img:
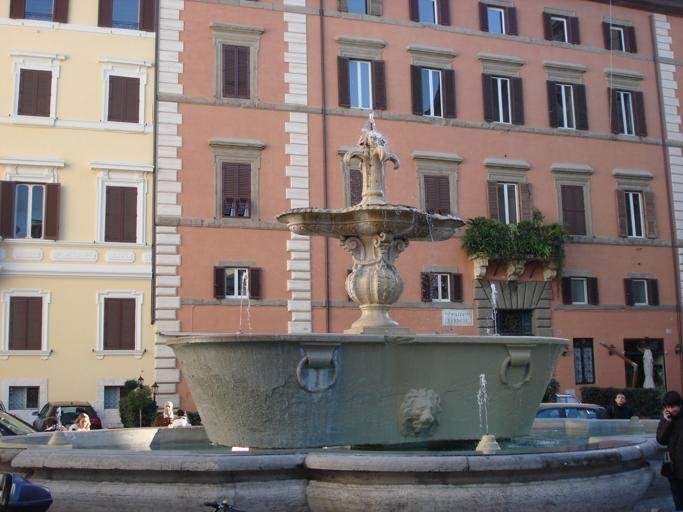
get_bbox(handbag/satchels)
[660,461,675,478]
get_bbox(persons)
[69,412,91,431]
[605,393,633,419]
[657,391,683,512]
[167,410,192,427]
[153,401,178,426]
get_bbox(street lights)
[151,380,158,404]
[137,374,146,427]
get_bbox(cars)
[554,393,581,402]
[534,402,608,419]
[31,401,100,430]
